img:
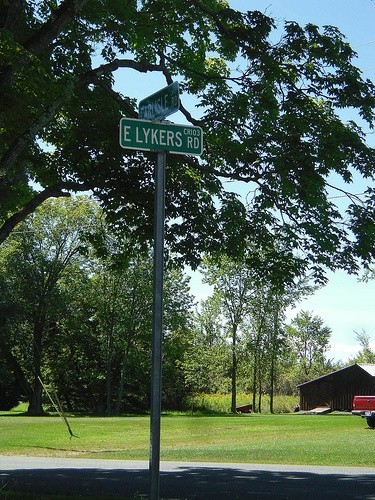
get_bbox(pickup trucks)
[352,394,375,427]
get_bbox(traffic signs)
[120,117,203,156]
[139,81,180,122]
